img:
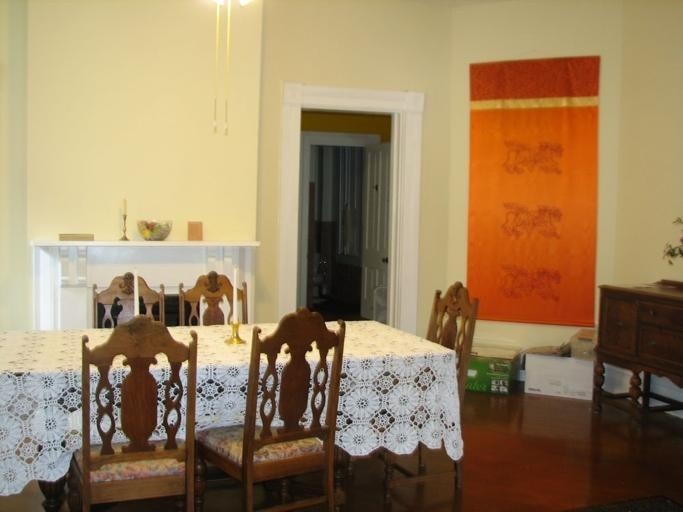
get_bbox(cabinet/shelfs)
[31,238,262,331]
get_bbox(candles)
[121,198,127,216]
[231,267,239,324]
[131,269,140,317]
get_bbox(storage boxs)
[568,326,597,361]
[466,345,520,397]
[523,345,594,400]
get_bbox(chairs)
[69,311,198,511]
[346,277,482,505]
[90,271,165,330]
[176,271,249,328]
[195,306,350,509]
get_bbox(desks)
[0,316,466,511]
[590,274,682,447]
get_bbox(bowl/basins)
[137,221,173,241]
